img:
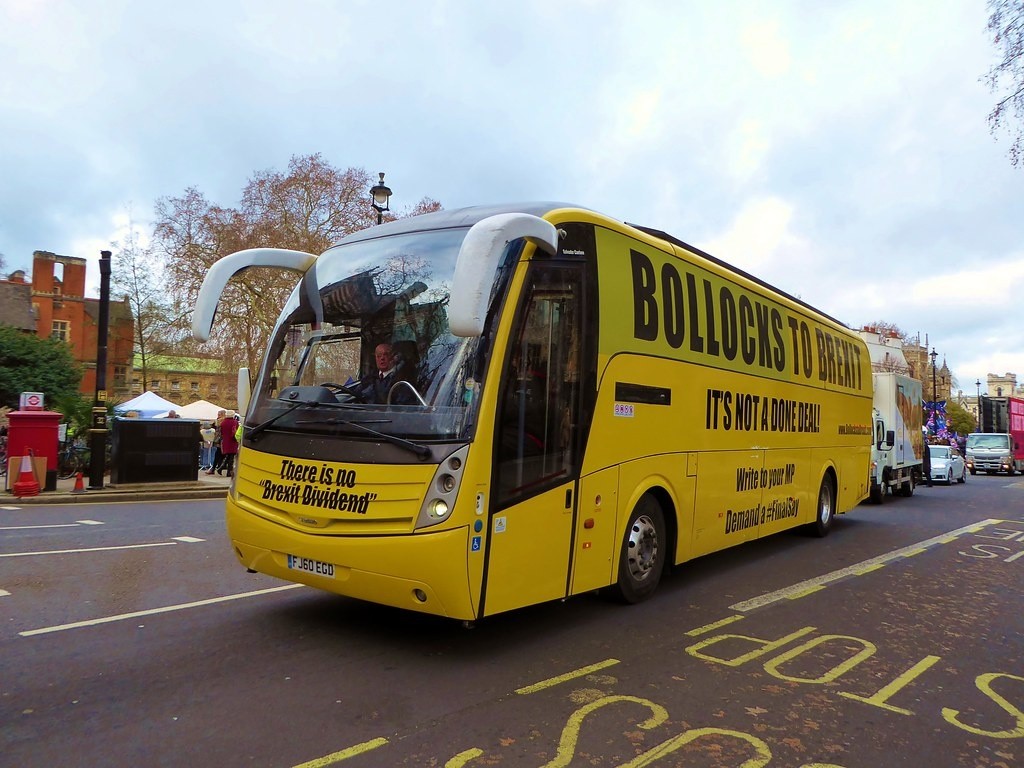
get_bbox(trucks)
[871,370,923,503]
[965,395,1024,477]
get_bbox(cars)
[918,444,967,485]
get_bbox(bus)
[187,198,877,629]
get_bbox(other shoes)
[228,473,233,477]
[923,484,933,488]
[205,467,215,474]
[217,469,223,475]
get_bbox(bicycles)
[56,441,90,480]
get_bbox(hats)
[225,410,235,417]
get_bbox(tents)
[106,391,181,423]
[152,399,240,420]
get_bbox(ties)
[380,372,384,381]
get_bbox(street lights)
[929,347,938,445]
[369,171,393,225]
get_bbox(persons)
[916,442,933,487]
[205,410,242,477]
[126,411,138,418]
[198,421,217,470]
[164,409,180,418]
[353,343,416,405]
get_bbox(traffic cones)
[12,445,39,496]
[72,473,87,492]
